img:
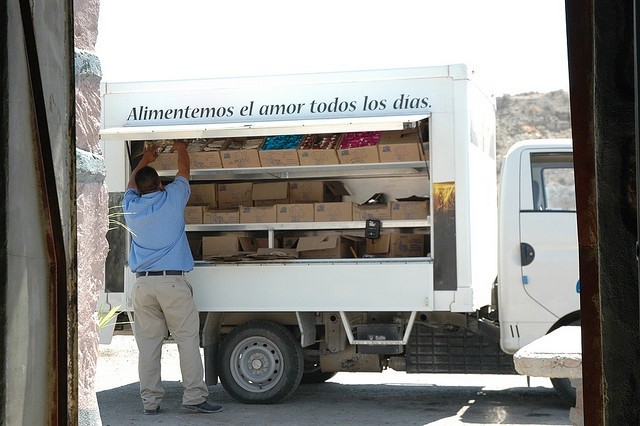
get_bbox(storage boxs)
[240,205,277,223]
[418,123,429,160]
[296,235,365,258]
[391,200,430,220]
[337,131,380,164]
[203,209,239,224]
[352,201,391,220]
[283,236,297,248]
[289,180,351,203]
[277,203,314,223]
[220,137,262,168]
[297,133,340,166]
[252,182,288,206]
[201,236,257,260]
[147,144,178,170]
[218,183,253,209]
[258,135,300,167]
[186,137,223,168]
[391,233,430,258]
[314,202,352,222]
[190,184,215,205]
[366,234,390,258]
[184,206,204,224]
[255,237,279,250]
[379,131,421,162]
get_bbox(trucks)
[100,64,580,407]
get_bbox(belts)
[137,270,183,276]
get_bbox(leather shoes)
[183,400,223,413]
[146,406,160,413]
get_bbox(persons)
[120,139,224,415]
